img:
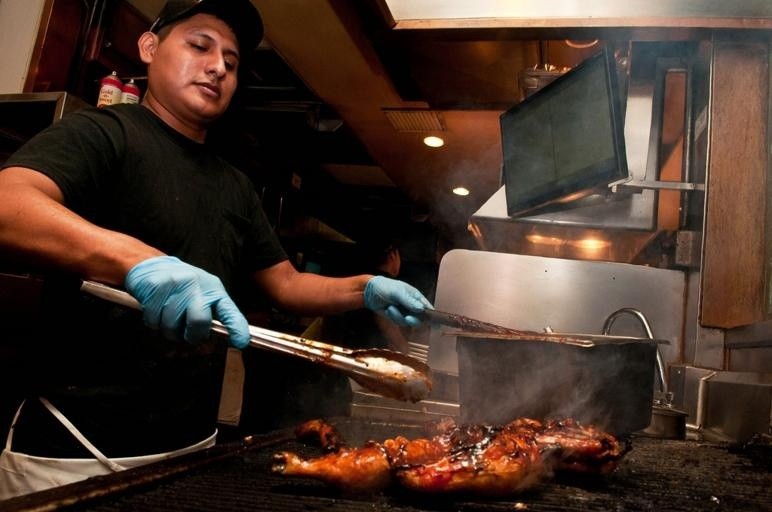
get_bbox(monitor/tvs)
[499,46,628,218]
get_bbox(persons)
[1,0,441,503]
[320,230,413,356]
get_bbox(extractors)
[467,41,657,265]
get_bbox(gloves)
[125,255,250,350]
[362,275,435,329]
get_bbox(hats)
[149,1,264,56]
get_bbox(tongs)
[76,273,435,405]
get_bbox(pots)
[442,324,670,444]
